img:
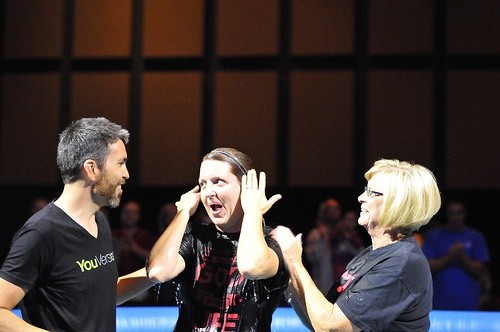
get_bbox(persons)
[268,156,441,332]
[0,117,158,332]
[145,147,291,332]
[26,198,492,313]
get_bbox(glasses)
[364,187,383,198]
